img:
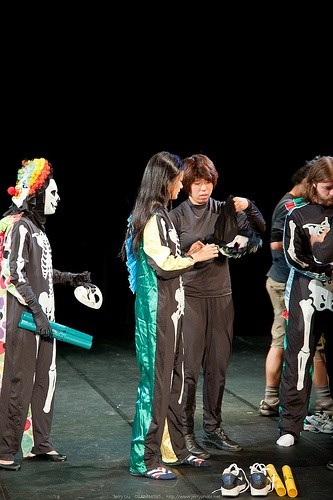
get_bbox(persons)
[276,157,333,447]
[257,156,333,419]
[167,152,269,465]
[120,150,212,482]
[0,153,95,472]
